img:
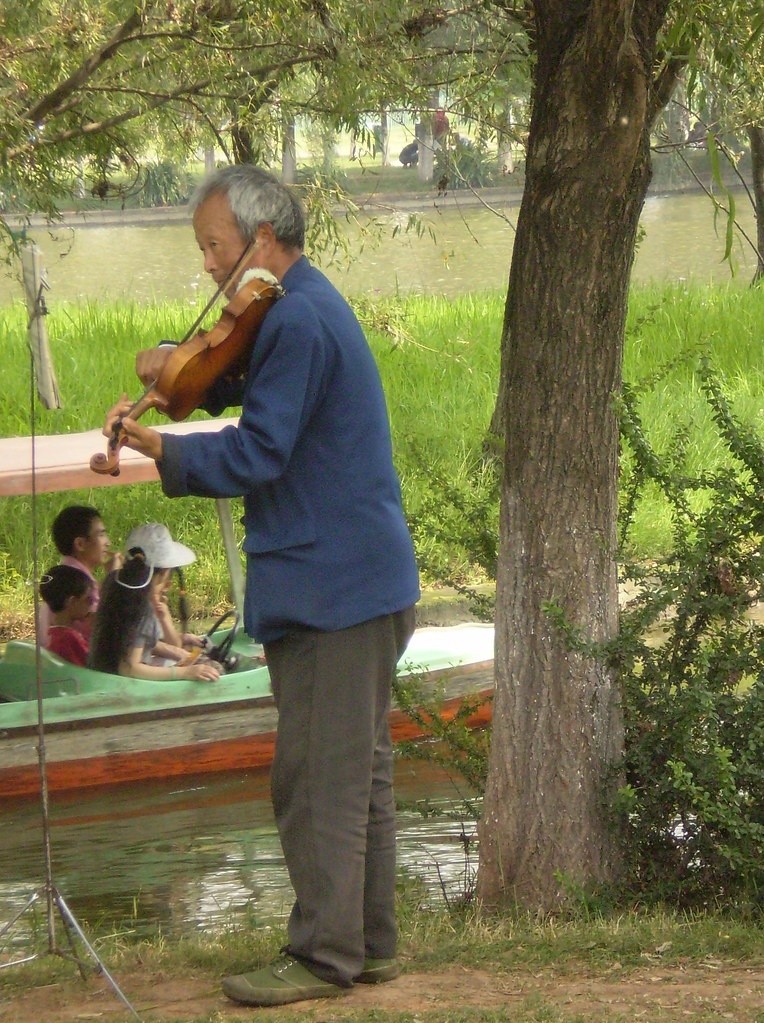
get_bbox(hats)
[125,523,195,569]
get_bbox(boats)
[0,417,495,796]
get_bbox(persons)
[398,139,419,169]
[39,506,224,684]
[104,164,421,1009]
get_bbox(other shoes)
[352,956,399,983]
[222,944,352,1004]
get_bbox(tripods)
[0,283,145,1023]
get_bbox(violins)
[88,277,281,479]
